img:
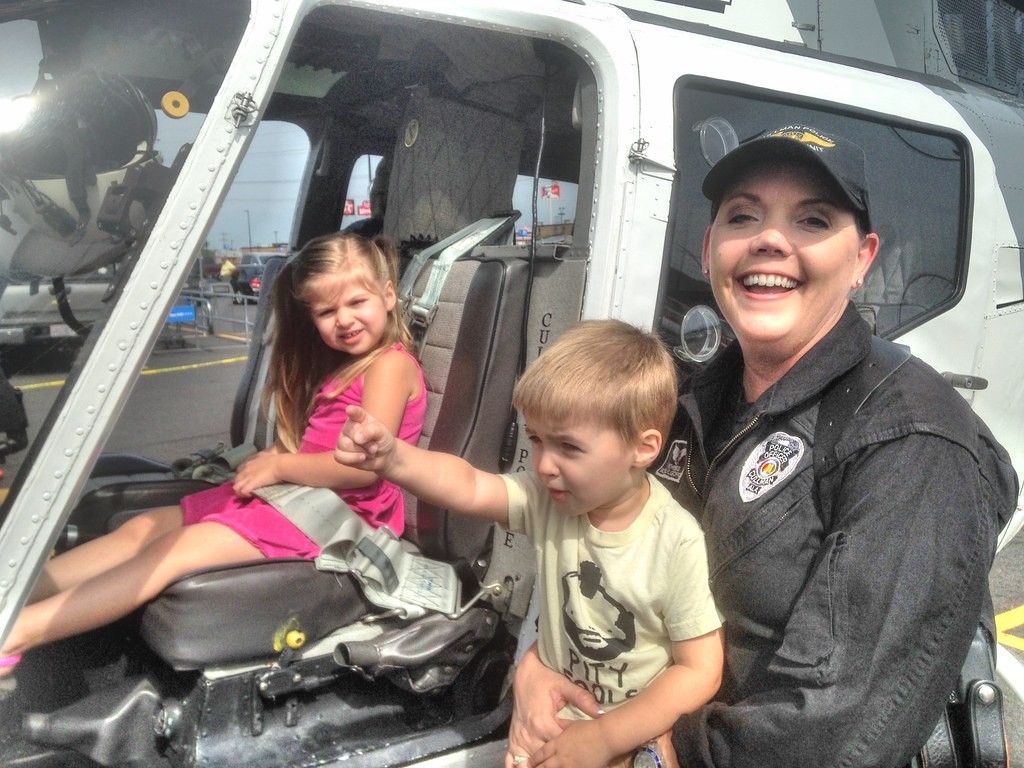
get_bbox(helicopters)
[0,0,1024,768]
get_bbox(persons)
[0,230,427,677]
[333,319,723,768]
[504,124,1020,768]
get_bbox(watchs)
[632,737,666,768]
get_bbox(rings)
[513,755,529,765]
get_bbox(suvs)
[231,253,282,304]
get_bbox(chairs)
[107,249,530,670]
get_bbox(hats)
[702,123,875,234]
